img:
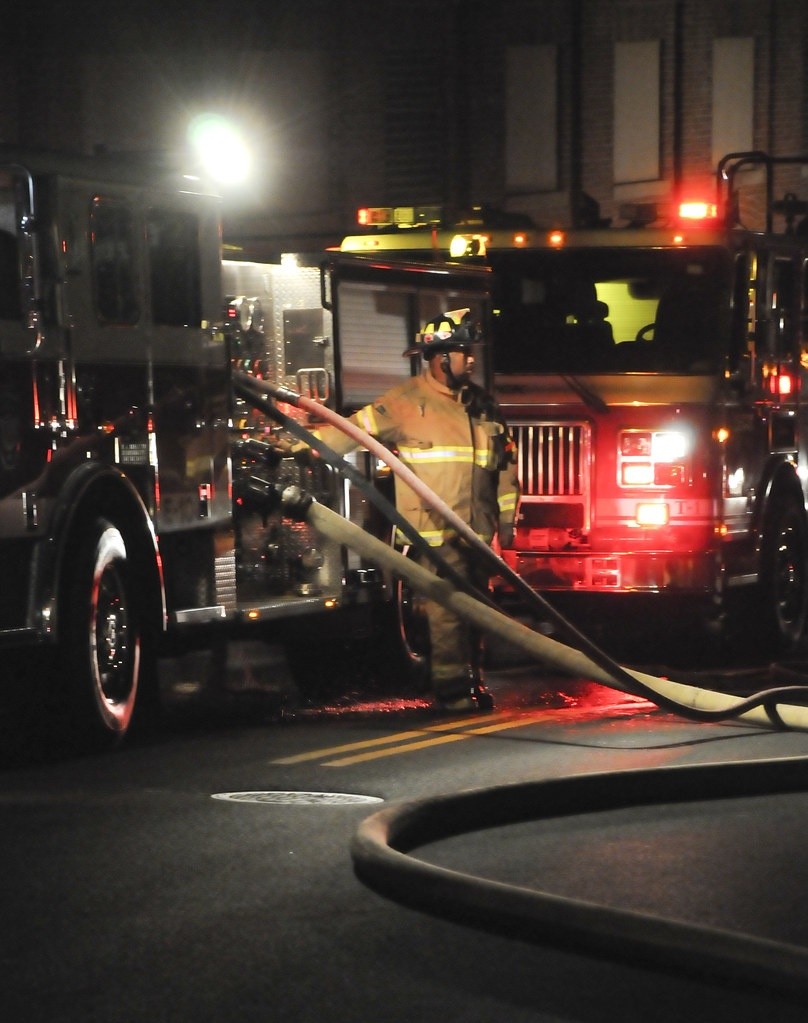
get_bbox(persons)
[276,308,521,714]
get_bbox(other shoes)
[473,676,496,711]
[432,688,474,714]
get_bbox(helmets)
[420,308,477,361]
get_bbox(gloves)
[498,522,513,550]
[267,438,293,458]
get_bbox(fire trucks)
[0,152,808,749]
